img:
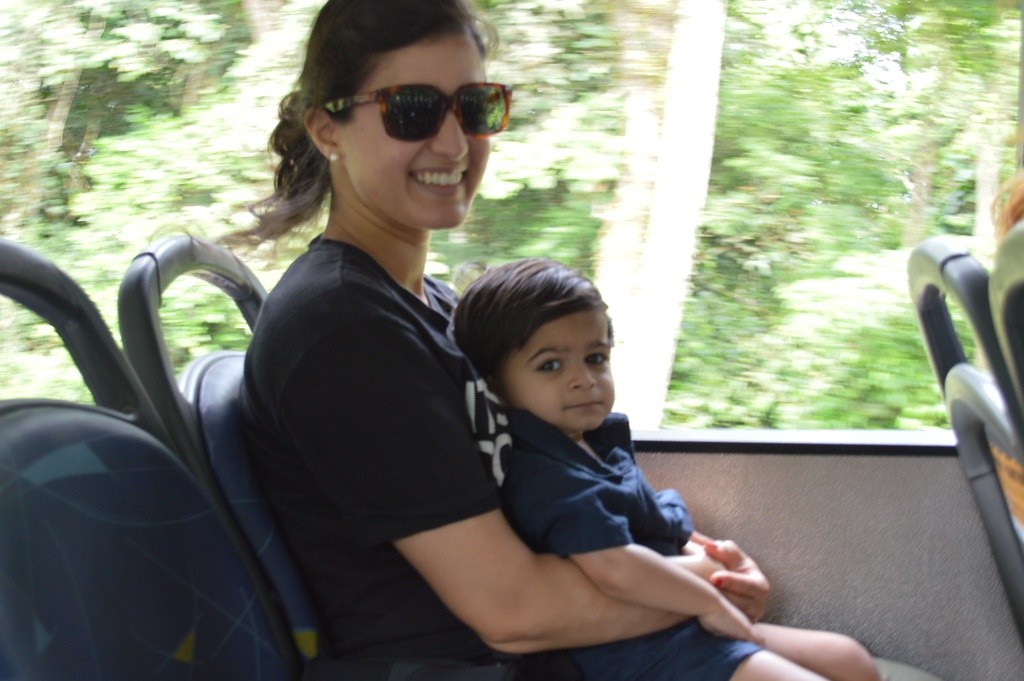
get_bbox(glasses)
[323,82,513,143]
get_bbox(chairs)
[117,235,334,681]
[905,232,1024,645]
[0,236,303,681]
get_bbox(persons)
[244,0,882,680]
